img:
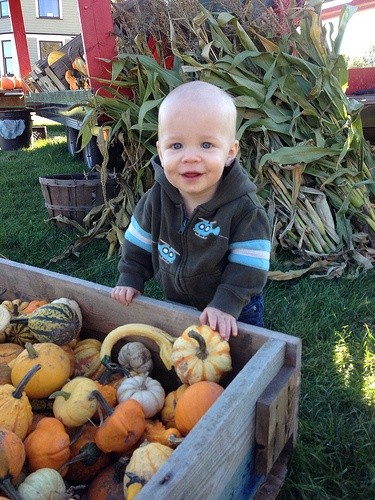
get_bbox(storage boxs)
[22,33,84,90]
[0,257,301,500]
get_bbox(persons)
[111,81,272,342]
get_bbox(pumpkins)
[0,298,232,500]
